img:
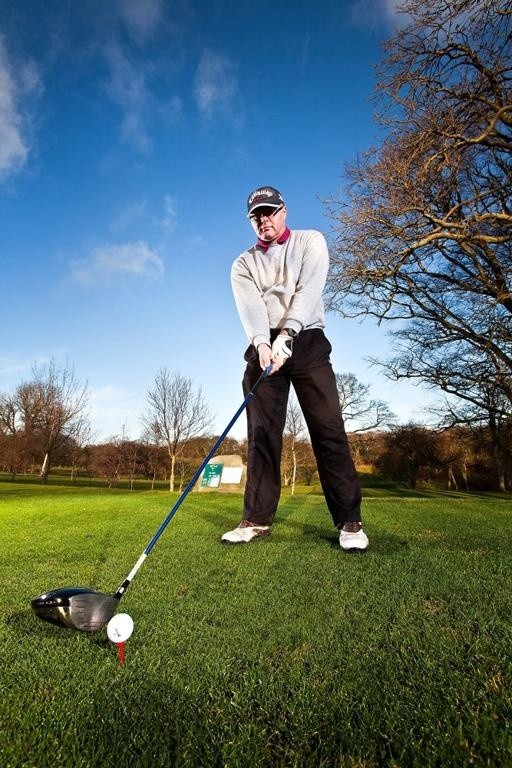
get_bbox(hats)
[247,187,283,218]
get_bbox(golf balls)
[106,613,134,643]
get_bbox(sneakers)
[221,520,271,545]
[338,521,368,551]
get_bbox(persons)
[220,185,371,551]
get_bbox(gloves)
[257,335,293,374]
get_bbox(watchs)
[284,328,297,339]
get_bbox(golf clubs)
[33,359,273,633]
